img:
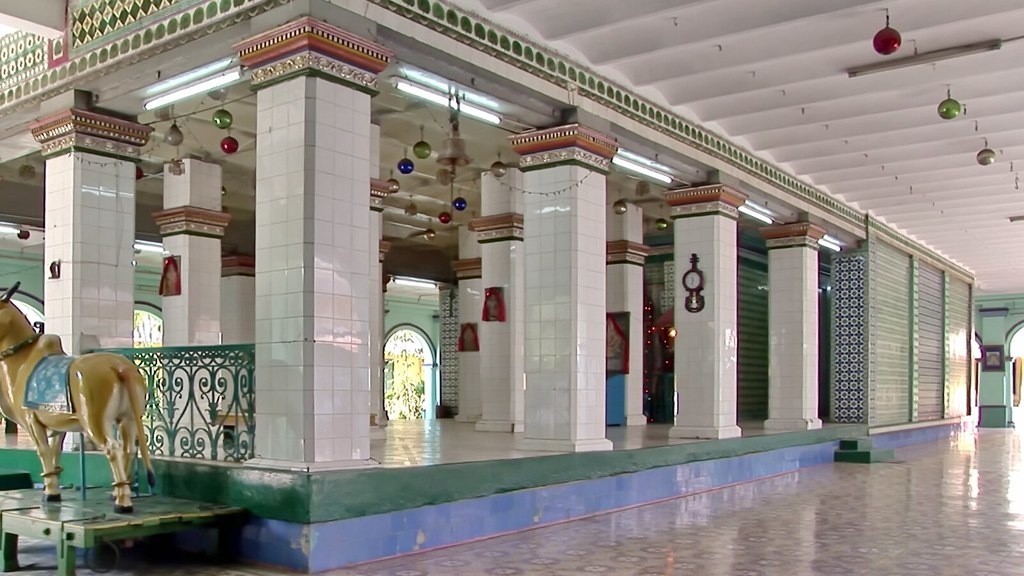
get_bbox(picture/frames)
[981,345,1006,372]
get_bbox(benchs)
[217,413,256,449]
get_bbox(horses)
[0,279,158,516]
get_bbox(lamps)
[142,65,242,111]
[612,189,668,230]
[937,87,961,119]
[738,205,775,224]
[847,38,1002,77]
[389,76,503,125]
[976,140,995,166]
[166,101,239,154]
[385,125,507,240]
[612,154,675,183]
[872,8,901,56]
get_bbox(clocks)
[682,253,705,313]
